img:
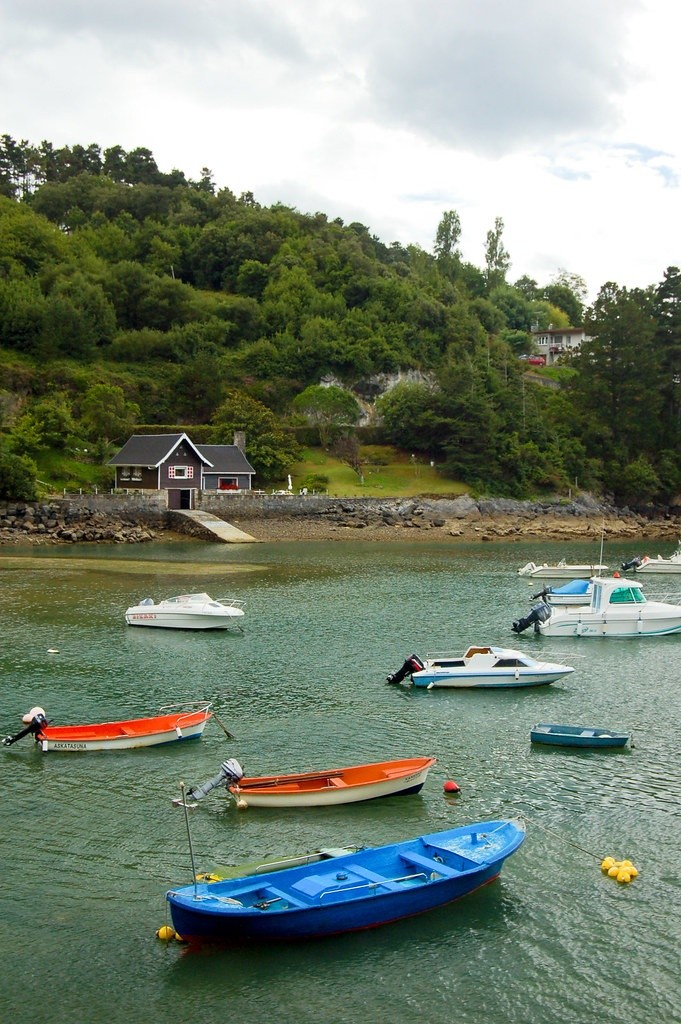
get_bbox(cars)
[519,354,546,366]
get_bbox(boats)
[1,699,215,753]
[530,722,629,748]
[512,576,681,638]
[528,517,641,605]
[387,646,578,688]
[125,593,246,632]
[186,756,438,810]
[620,540,681,573]
[517,557,608,578]
[165,817,526,949]
